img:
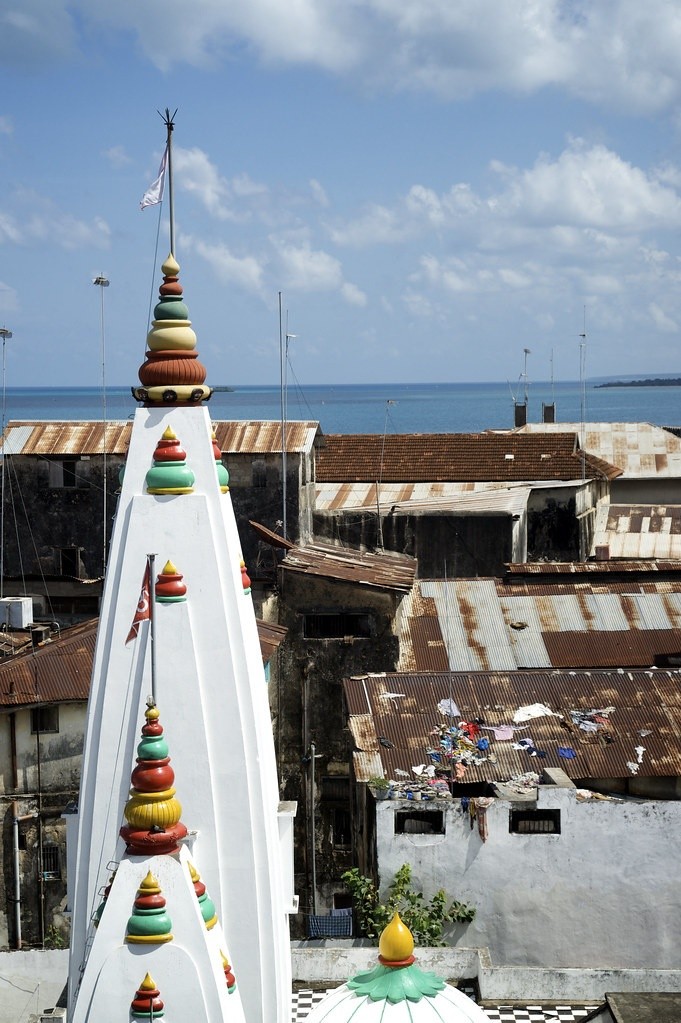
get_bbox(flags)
[126,559,152,643]
[140,149,167,209]
